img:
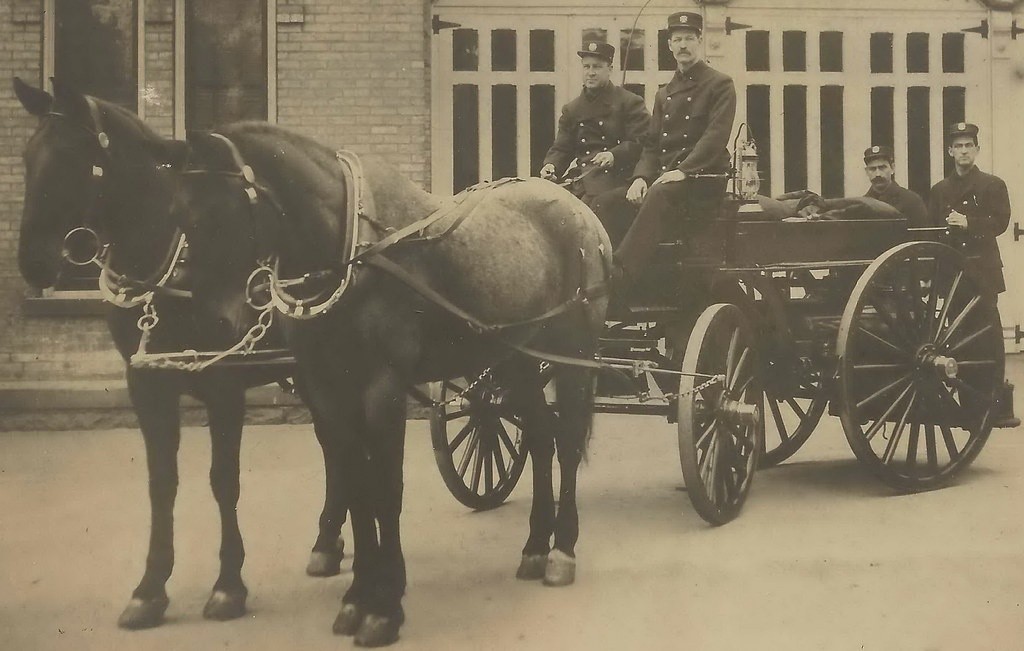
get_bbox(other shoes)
[612,261,638,298]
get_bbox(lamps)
[733,123,761,201]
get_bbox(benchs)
[632,194,742,266]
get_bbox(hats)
[864,146,893,165]
[577,40,615,64]
[667,12,703,35]
[949,122,979,137]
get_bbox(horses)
[143,120,615,648]
[8,72,345,631]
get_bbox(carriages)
[10,73,1024,646]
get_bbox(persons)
[539,41,653,251]
[590,12,737,287]
[862,123,1011,407]
